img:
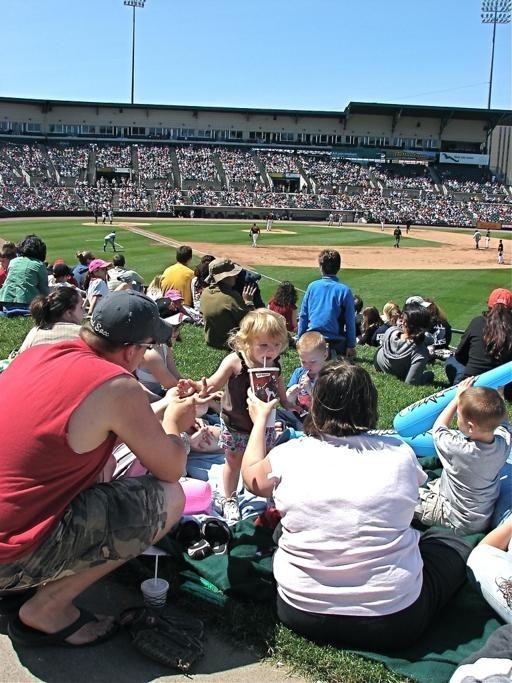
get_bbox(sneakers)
[219,497,242,520]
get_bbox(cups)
[139,578,169,611]
[248,367,279,428]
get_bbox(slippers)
[3,602,118,646]
[176,515,234,557]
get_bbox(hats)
[90,290,186,344]
[202,256,246,283]
[487,285,512,308]
[52,264,72,277]
[405,293,434,308]
[87,260,114,272]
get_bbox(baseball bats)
[114,242,124,249]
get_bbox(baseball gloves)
[120,604,205,673]
